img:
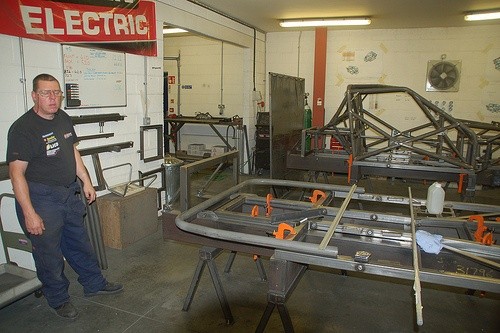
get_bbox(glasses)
[36,90,63,97]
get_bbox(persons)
[7,74,124,320]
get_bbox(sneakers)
[84,282,124,296]
[48,301,79,320]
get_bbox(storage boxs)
[211,146,228,157]
[187,143,204,155]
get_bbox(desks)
[164,116,243,170]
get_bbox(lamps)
[280,17,372,27]
[464,10,500,21]
[163,26,189,34]
